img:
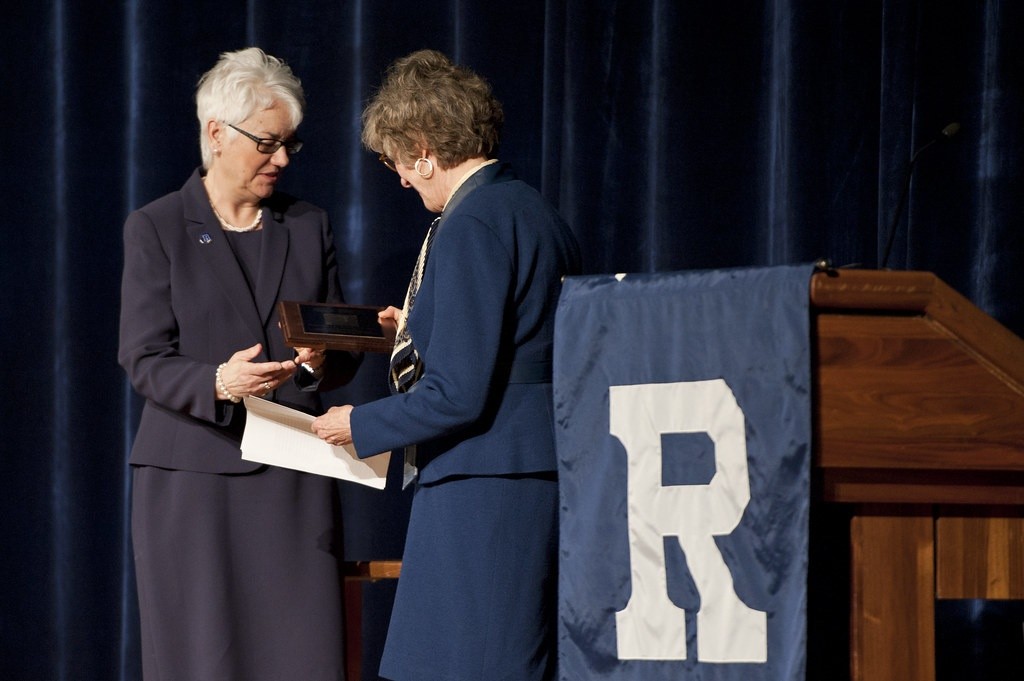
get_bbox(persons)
[112,46,367,681]
[309,51,572,681]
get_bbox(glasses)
[378,152,399,172]
[227,124,303,154]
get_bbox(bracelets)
[215,362,245,403]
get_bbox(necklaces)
[200,177,264,232]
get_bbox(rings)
[263,382,274,391]
